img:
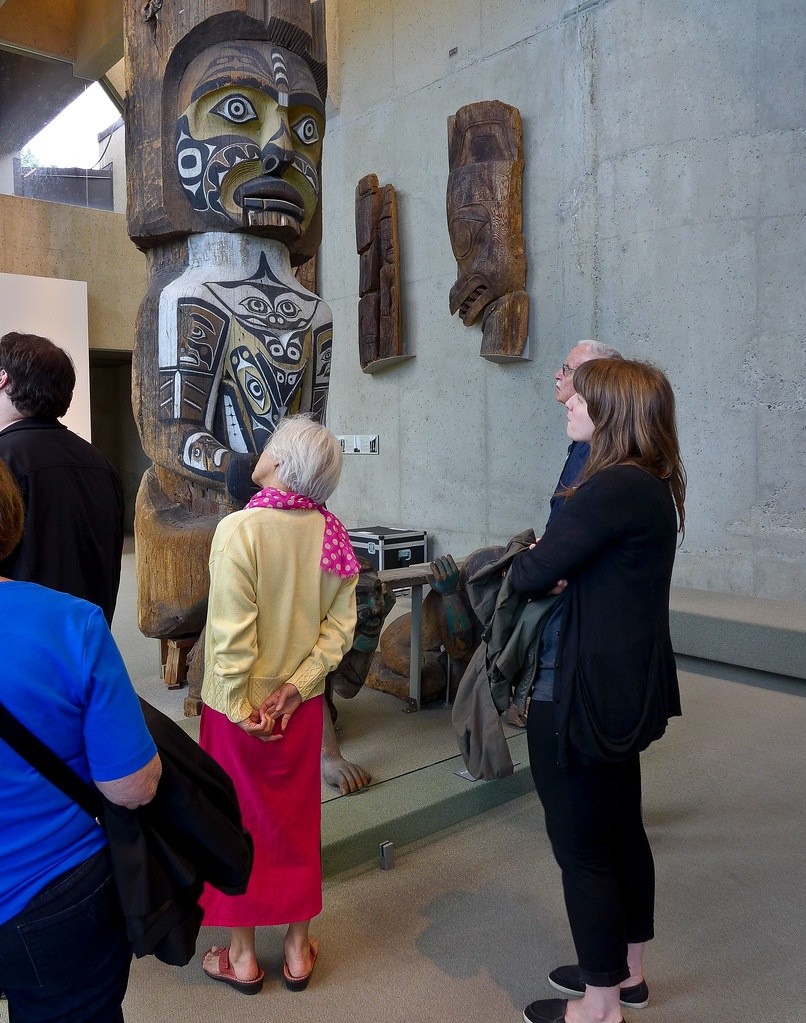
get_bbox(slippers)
[201,945,265,995]
[283,934,319,992]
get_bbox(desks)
[380,568,464,710]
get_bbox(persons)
[198,420,359,996]
[125,0,373,795]
[545,339,623,504]
[0,331,126,628]
[0,462,163,1023]
[511,359,684,1022]
[364,545,506,704]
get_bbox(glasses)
[562,364,576,376]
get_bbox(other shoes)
[548,965,650,1008]
[523,998,626,1023]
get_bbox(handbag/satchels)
[108,810,205,969]
